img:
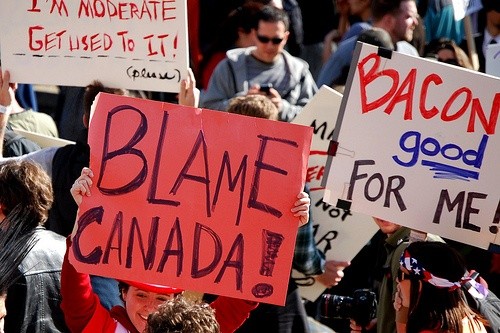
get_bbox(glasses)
[256,32,285,46]
[394,14,419,21]
[436,56,456,65]
[396,268,410,282]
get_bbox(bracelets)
[0,105,12,114]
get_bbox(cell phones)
[260,84,272,97]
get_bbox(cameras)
[317,289,377,328]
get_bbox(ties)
[489,38,498,44]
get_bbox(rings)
[76,180,80,184]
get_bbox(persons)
[0,68,200,235]
[6,83,59,138]
[61,167,311,333]
[227,96,351,333]
[202,7,318,123]
[394,242,488,333]
[142,294,220,333]
[0,160,71,333]
[223,0,500,89]
